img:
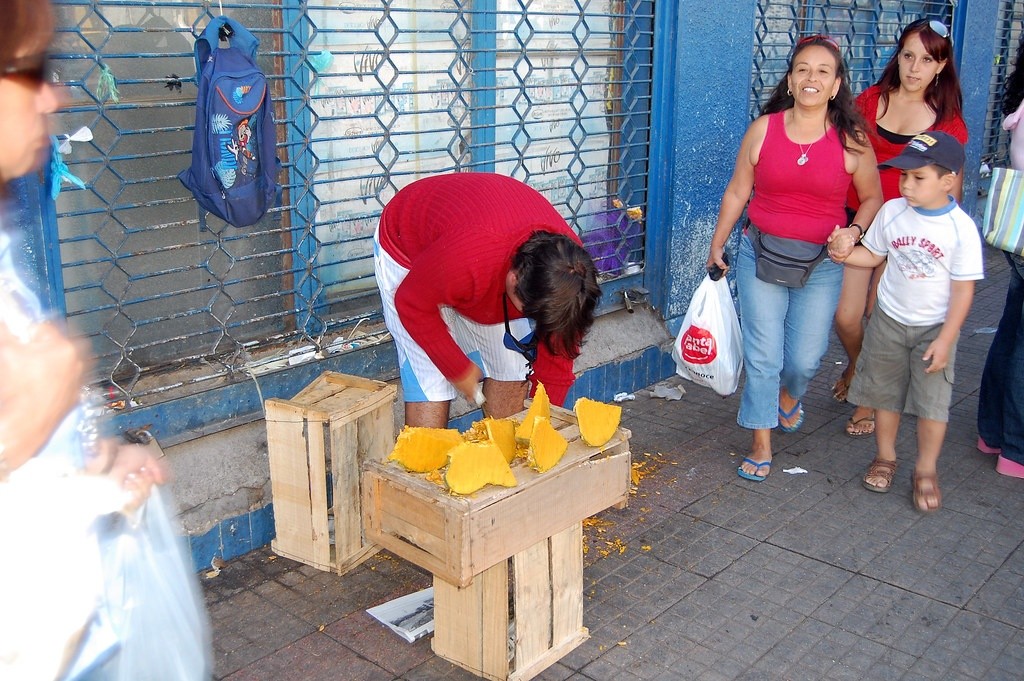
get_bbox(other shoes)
[977,436,1001,454]
[995,454,1024,478]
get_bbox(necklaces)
[794,127,821,165]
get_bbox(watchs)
[848,224,864,243]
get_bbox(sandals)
[911,466,942,512]
[862,459,897,493]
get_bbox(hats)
[877,130,965,174]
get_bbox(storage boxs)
[431,522,590,681]
[265,370,397,576]
[361,397,632,589]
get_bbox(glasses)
[0,50,48,90]
[904,19,950,38]
[799,36,839,53]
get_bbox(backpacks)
[176,15,282,228]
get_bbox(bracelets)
[98,440,118,475]
[0,444,12,484]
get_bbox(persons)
[706,33,884,482]
[372,173,602,431]
[830,18,966,436]
[0,0,165,681]
[825,130,983,508]
[976,44,1024,479]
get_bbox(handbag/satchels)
[746,223,830,288]
[982,167,1024,257]
[120,468,209,681]
[671,273,743,396]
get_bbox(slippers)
[738,458,771,481]
[778,401,805,433]
[846,417,875,439]
[835,371,849,402]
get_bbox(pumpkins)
[528,416,569,472]
[514,380,550,446]
[483,417,516,464]
[388,424,464,472]
[444,442,517,494]
[574,396,622,445]
